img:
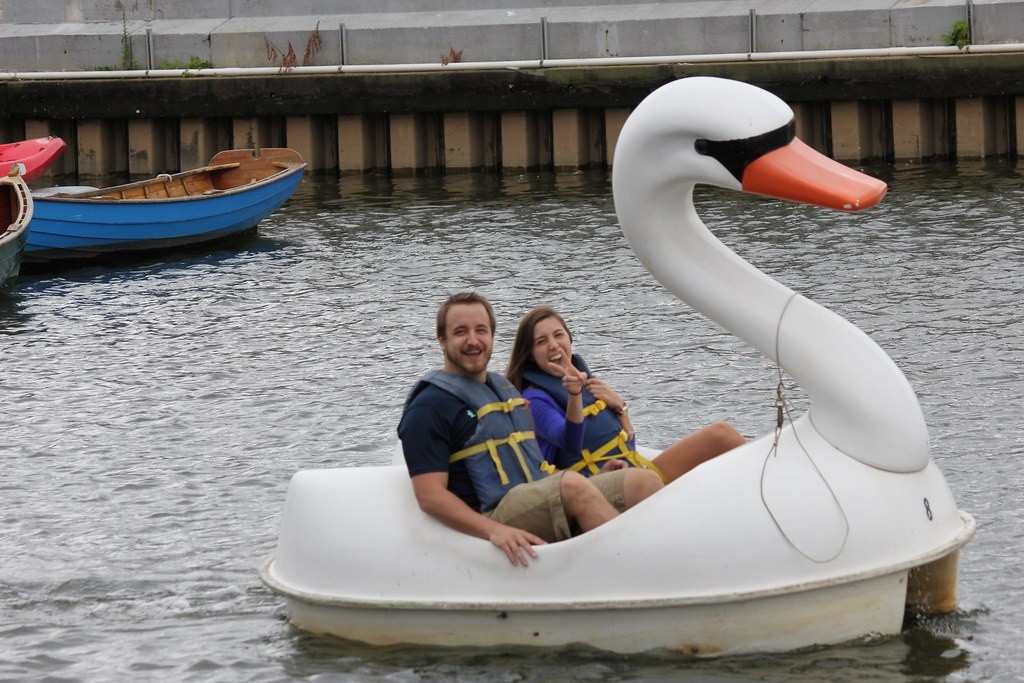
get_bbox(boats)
[0,163,34,287]
[21,146,308,253]
[258,75,978,655]
[0,135,66,185]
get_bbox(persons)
[506,306,748,486]
[396,292,665,567]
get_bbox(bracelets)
[568,388,582,395]
[618,402,629,416]
